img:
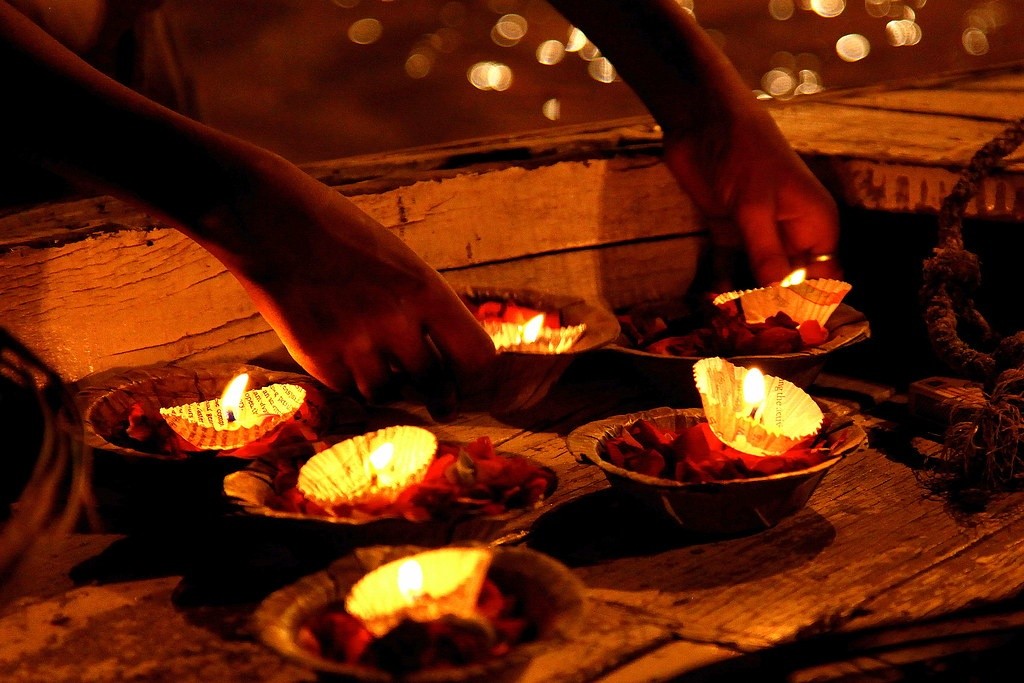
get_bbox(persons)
[0,2,845,404]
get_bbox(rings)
[807,253,839,263]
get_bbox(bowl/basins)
[597,299,871,393]
[399,286,620,414]
[57,360,298,465]
[220,435,560,548]
[564,407,867,528]
[250,544,588,683]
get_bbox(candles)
[738,408,762,424]
[364,472,392,491]
[407,591,433,608]
[221,408,239,431]
